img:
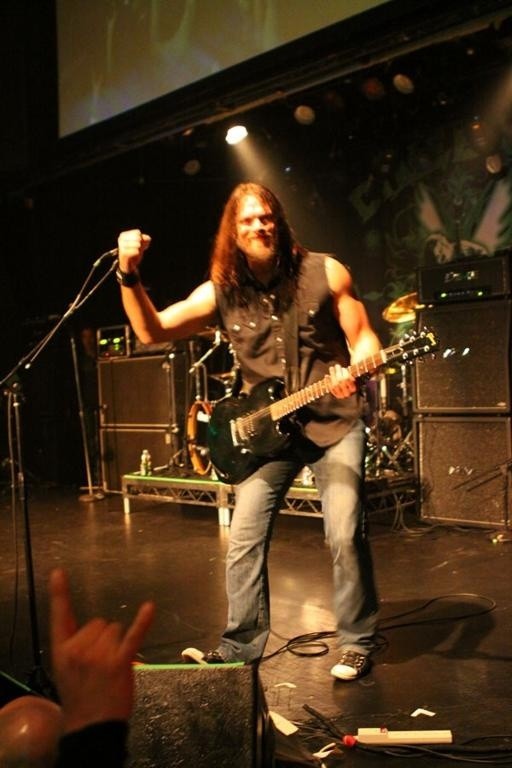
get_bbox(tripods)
[363,365,415,476]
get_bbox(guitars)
[207,329,440,485]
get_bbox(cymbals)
[382,292,419,323]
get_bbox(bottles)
[139,449,152,477]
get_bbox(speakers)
[99,427,186,494]
[96,353,187,428]
[128,661,277,768]
[411,416,512,532]
[410,299,511,413]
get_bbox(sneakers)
[330,650,374,681]
[180,645,226,665]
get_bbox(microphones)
[91,404,108,410]
[215,324,221,345]
[196,447,210,457]
[101,242,150,257]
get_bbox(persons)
[48,567,157,768]
[0,695,64,768]
[110,179,386,682]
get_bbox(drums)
[377,366,410,421]
[185,400,226,476]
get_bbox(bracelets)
[114,268,141,287]
